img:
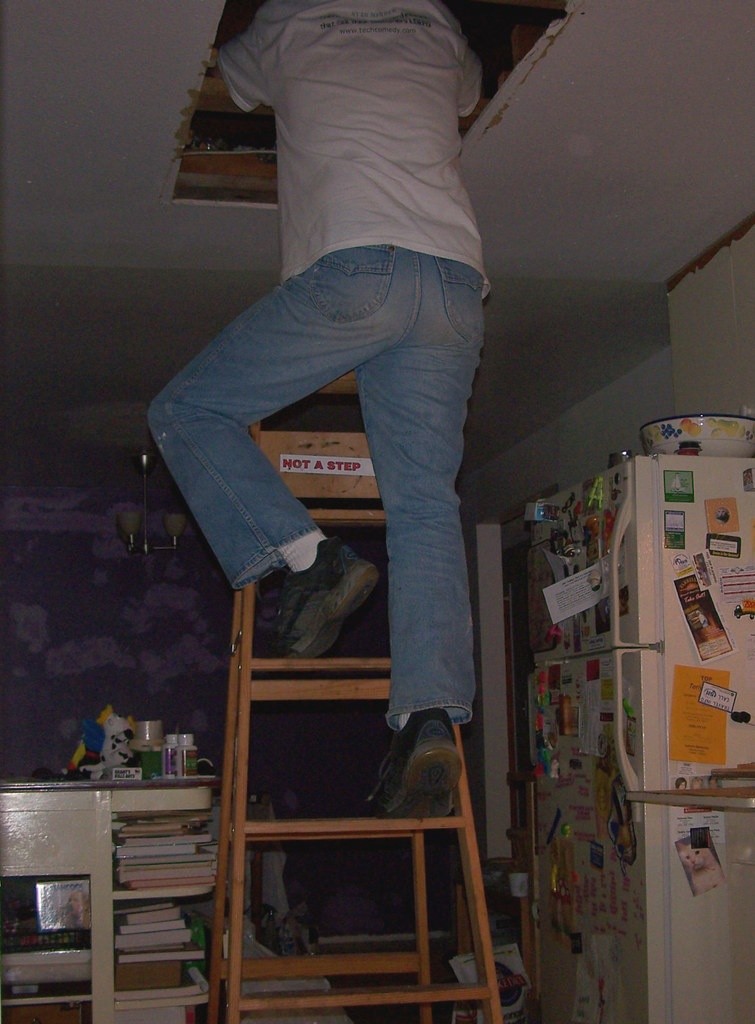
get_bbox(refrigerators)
[525,452,755,1023]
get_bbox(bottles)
[161,733,199,780]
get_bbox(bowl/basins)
[639,414,755,456]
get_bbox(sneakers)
[364,708,461,848]
[262,537,379,660]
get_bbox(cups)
[608,449,632,468]
[509,871,529,899]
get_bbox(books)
[112,817,218,1024]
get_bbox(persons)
[148,0,494,821]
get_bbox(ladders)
[207,366,510,1024]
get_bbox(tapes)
[133,720,164,740]
